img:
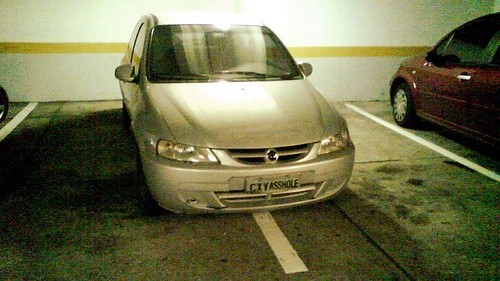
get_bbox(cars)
[114,12,354,214]
[390,12,500,163]
